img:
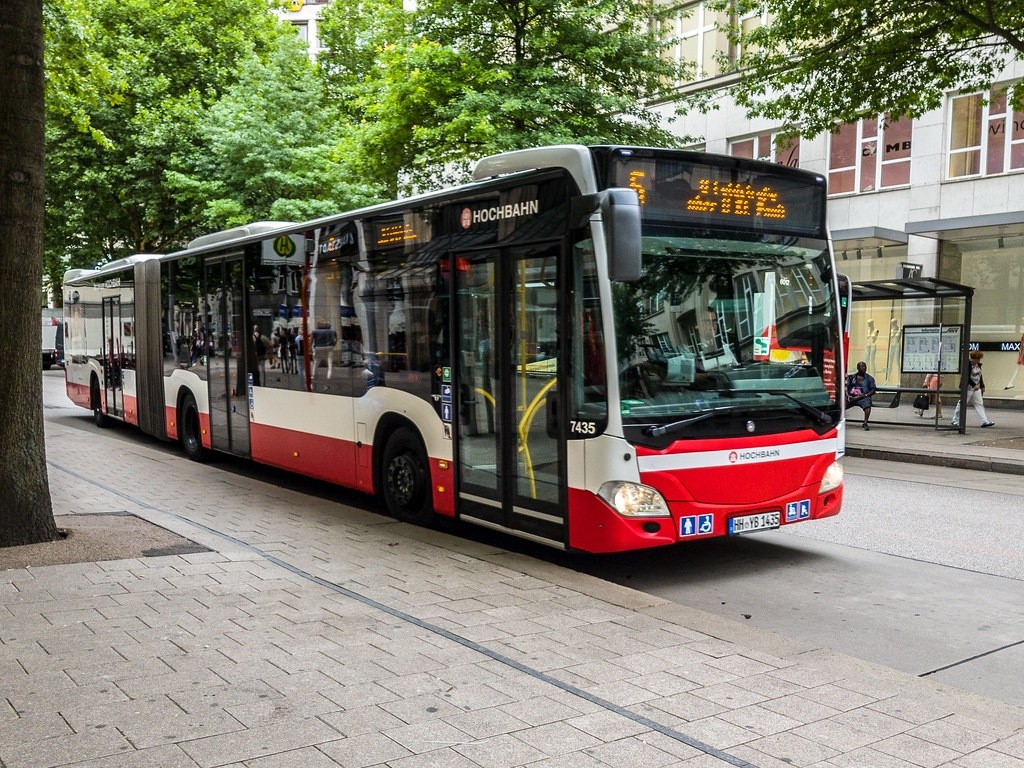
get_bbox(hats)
[254,325,260,332]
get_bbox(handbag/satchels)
[849,387,863,397]
[914,393,929,410]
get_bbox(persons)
[695,307,743,370]
[863,318,901,386]
[181,317,338,385]
[844,361,877,431]
[951,350,995,430]
[1003,332,1024,389]
[582,314,603,386]
[912,372,943,421]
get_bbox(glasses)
[703,319,718,326]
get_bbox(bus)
[63,144,852,552]
[753,264,852,405]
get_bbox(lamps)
[856,249,861,260]
[998,237,1005,249]
[877,247,882,258]
[841,251,848,261]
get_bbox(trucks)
[41,309,64,370]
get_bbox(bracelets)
[865,394,868,397]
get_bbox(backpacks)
[254,334,266,356]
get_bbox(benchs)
[870,389,901,408]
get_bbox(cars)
[54,322,64,366]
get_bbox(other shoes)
[291,370,298,375]
[271,366,275,369]
[282,368,285,374]
[981,422,994,427]
[862,423,869,430]
[286,367,289,373]
[278,363,280,369]
[931,415,942,420]
[914,409,923,416]
[951,422,960,427]
